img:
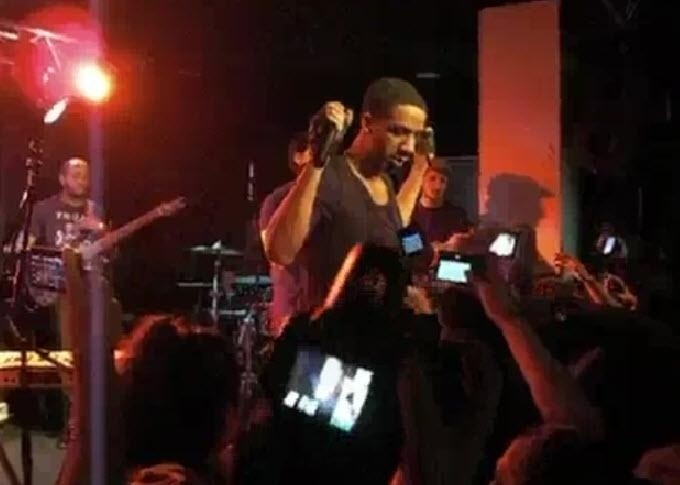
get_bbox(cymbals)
[177,282,222,288]
[182,246,244,256]
[226,275,271,285]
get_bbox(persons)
[8,154,123,449]
[57,78,680,485]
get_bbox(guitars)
[16,196,187,313]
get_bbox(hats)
[431,159,450,174]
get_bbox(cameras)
[437,250,476,287]
[597,227,619,259]
[397,224,424,255]
[487,227,519,261]
[286,338,376,438]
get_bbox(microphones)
[246,156,256,201]
